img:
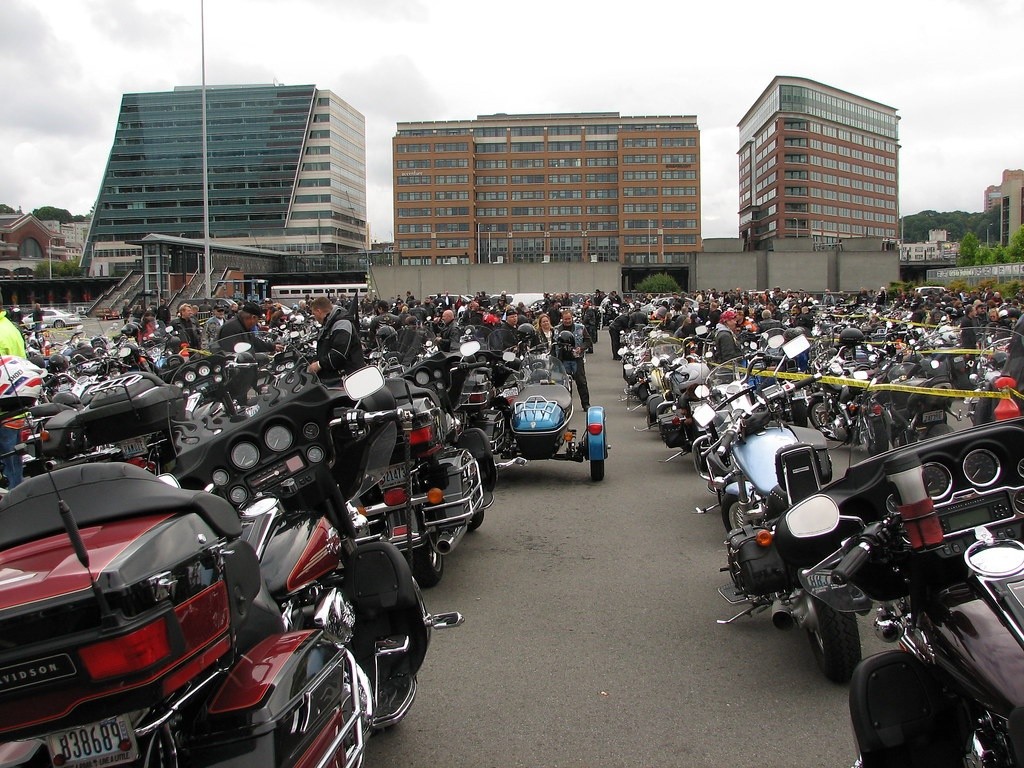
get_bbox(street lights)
[48,237,56,279]
[793,218,798,238]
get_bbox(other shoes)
[586,350,593,353]
[584,405,591,411]
[613,356,622,360]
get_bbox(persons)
[0,281,1024,491]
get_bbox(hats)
[214,305,224,310]
[243,302,261,317]
[506,309,518,320]
[719,311,737,324]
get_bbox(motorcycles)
[623,294,1024,767]
[1,313,613,768]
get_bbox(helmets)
[498,295,507,301]
[46,354,69,373]
[783,326,805,344]
[120,323,139,337]
[839,328,865,345]
[376,326,398,347]
[0,355,48,413]
[518,323,536,339]
[557,329,576,352]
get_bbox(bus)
[271,284,367,310]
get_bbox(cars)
[915,287,945,302]
[19,310,82,330]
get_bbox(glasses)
[981,308,986,309]
[217,310,224,312]
